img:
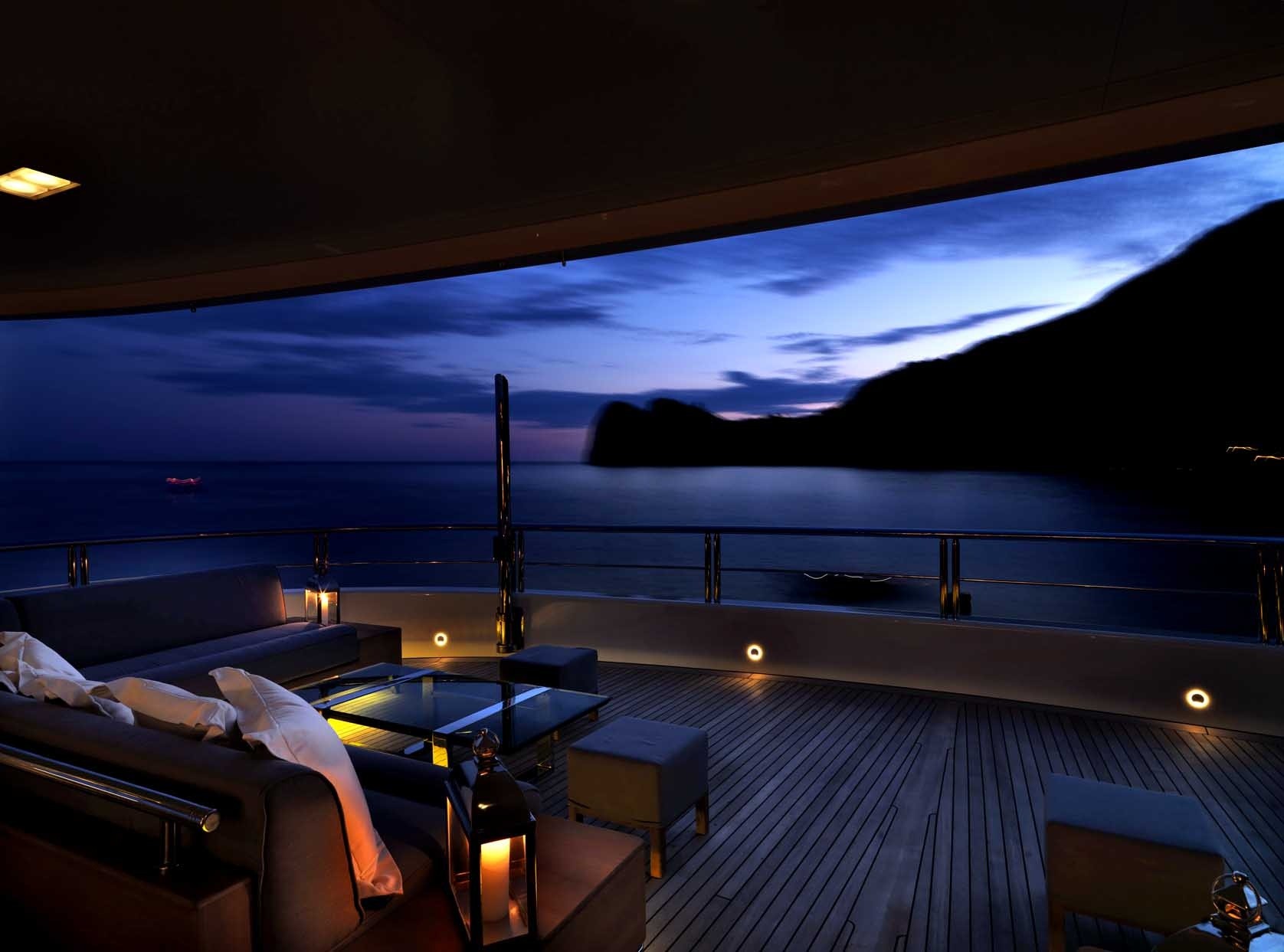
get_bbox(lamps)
[443,730,538,951]
[306,566,342,628]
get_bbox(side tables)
[290,617,402,669]
[344,813,645,951]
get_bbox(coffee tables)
[297,664,610,775]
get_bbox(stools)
[500,646,600,741]
[1043,771,1227,952]
[565,715,709,879]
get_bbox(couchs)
[0,565,356,709]
[0,685,540,952]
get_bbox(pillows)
[90,674,235,742]
[1,630,94,707]
[205,668,403,900]
